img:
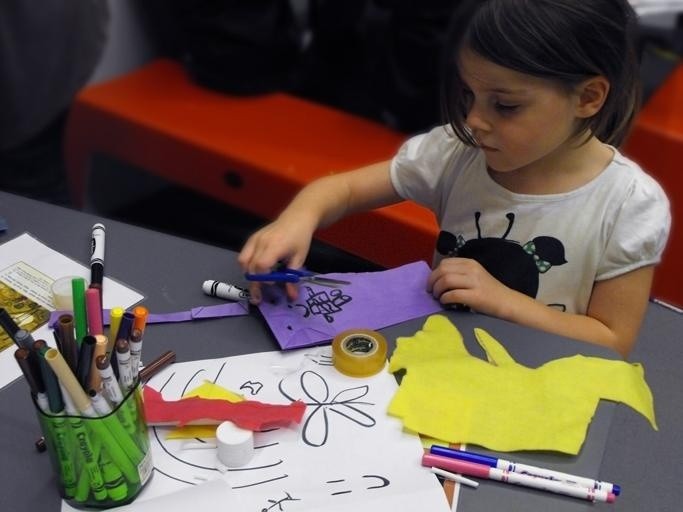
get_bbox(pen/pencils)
[2,219,149,498]
[420,442,622,506]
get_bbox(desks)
[610,64,682,309]
[1,186,683,512]
[63,53,446,274]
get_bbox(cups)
[29,375,154,509]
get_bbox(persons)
[238,0,672,362]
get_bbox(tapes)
[332,325,389,377]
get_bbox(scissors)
[241,263,352,299]
